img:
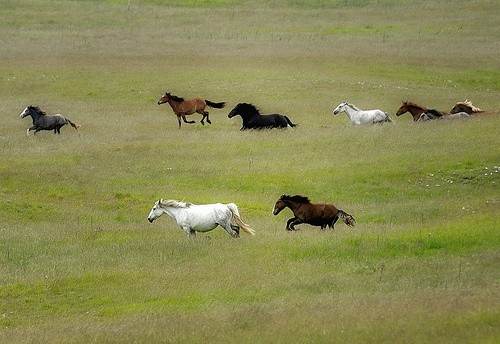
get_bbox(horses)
[148,199,256,238]
[20,105,78,135]
[228,103,297,131]
[157,92,225,130]
[396,98,486,122]
[273,194,357,231]
[333,103,392,124]
